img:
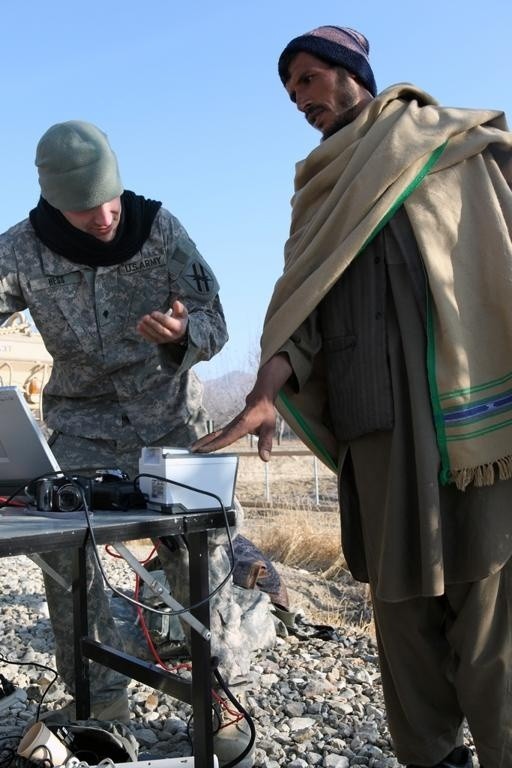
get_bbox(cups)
[16,720,77,768]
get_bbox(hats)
[277,24,377,96]
[34,120,123,213]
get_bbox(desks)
[0,503,235,768]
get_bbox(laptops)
[0,386,122,496]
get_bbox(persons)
[2,117,258,767]
[187,23,509,768]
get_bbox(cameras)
[36,474,92,512]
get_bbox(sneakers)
[34,692,130,726]
[215,696,255,768]
